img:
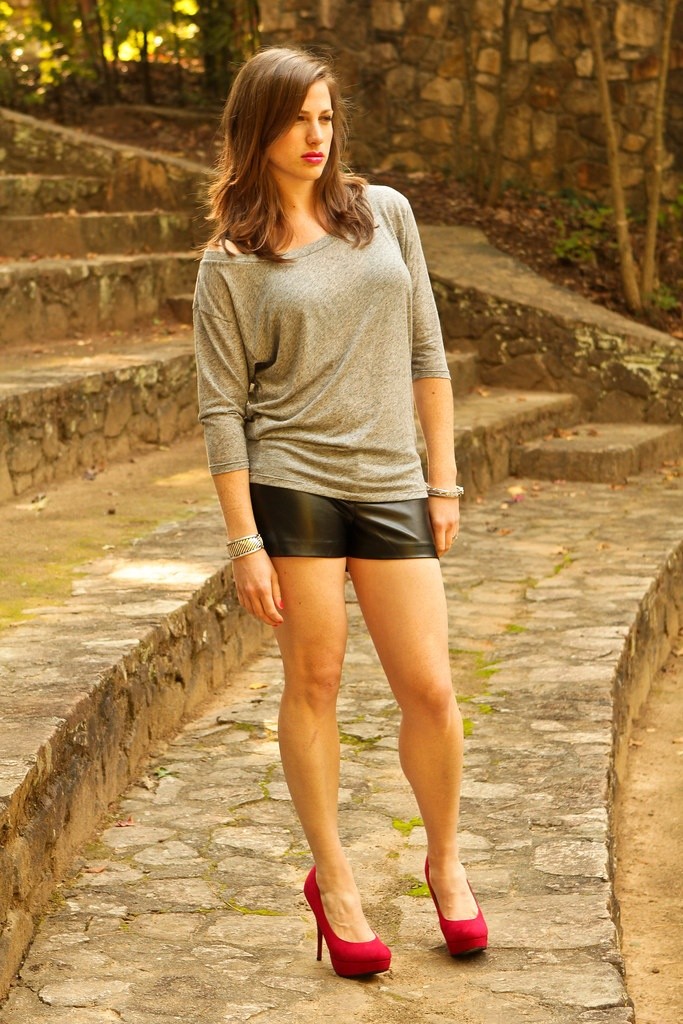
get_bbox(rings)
[453,536,458,540]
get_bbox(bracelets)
[426,482,465,497]
[227,534,264,560]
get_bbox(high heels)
[304,865,392,977]
[425,856,489,955]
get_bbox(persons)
[193,48,488,976]
